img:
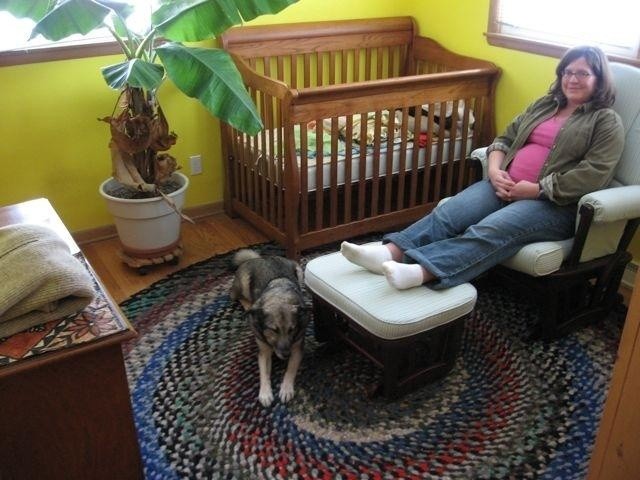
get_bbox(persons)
[339,45,627,290]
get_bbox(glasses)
[560,69,594,80]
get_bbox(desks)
[1,198,151,479]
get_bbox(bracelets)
[536,181,545,201]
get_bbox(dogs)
[228,247,312,410]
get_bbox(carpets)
[114,234,618,480]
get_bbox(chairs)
[432,60,640,340]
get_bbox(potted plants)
[1,0,301,265]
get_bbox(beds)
[219,11,502,253]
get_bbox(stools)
[303,242,476,395]
